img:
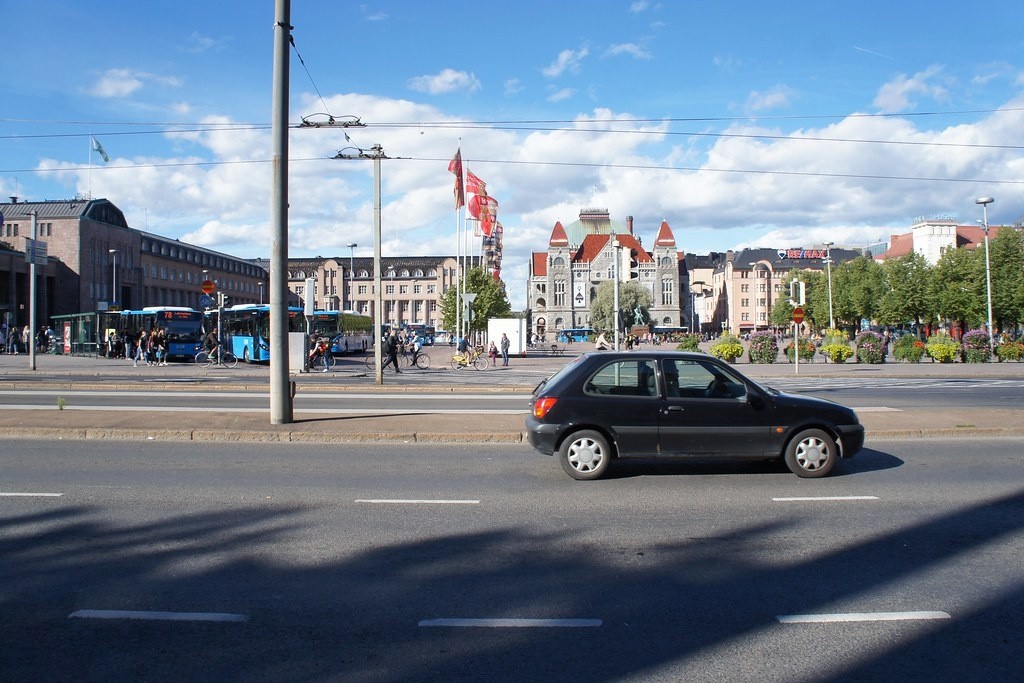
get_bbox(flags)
[92,136,109,162]
[447,149,503,268]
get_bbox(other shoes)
[410,362,415,366]
[208,354,215,359]
[322,369,329,372]
[502,364,508,366]
[467,364,471,366]
[396,371,402,373]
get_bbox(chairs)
[639,366,651,394]
[645,368,657,396]
[541,342,567,357]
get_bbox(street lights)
[388,266,395,331]
[257,281,265,303]
[108,249,120,305]
[346,243,357,311]
[203,270,210,280]
[975,196,996,356]
[822,240,835,331]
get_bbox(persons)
[596,331,612,350]
[408,332,422,366]
[37,326,54,353]
[22,325,30,354]
[8,327,19,356]
[429,334,434,348]
[501,333,510,366]
[133,330,169,367]
[109,330,132,360]
[382,329,403,374]
[310,329,329,372]
[457,334,475,366]
[527,332,537,349]
[811,333,822,346]
[634,304,645,325]
[490,341,497,366]
[745,332,750,346]
[448,334,453,349]
[643,332,671,345]
[779,332,784,343]
[883,330,890,358]
[625,332,639,350]
[204,328,221,358]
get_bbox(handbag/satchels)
[457,347,460,351]
[383,341,390,353]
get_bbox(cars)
[524,350,865,481]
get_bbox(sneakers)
[133,361,168,367]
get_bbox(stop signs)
[792,307,806,324]
[201,280,215,294]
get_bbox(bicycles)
[311,350,336,369]
[451,347,489,371]
[365,344,431,370]
[195,344,238,369]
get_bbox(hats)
[412,331,418,334]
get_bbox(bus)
[373,323,449,345]
[314,310,373,356]
[560,328,595,343]
[205,304,305,364]
[121,306,202,362]
